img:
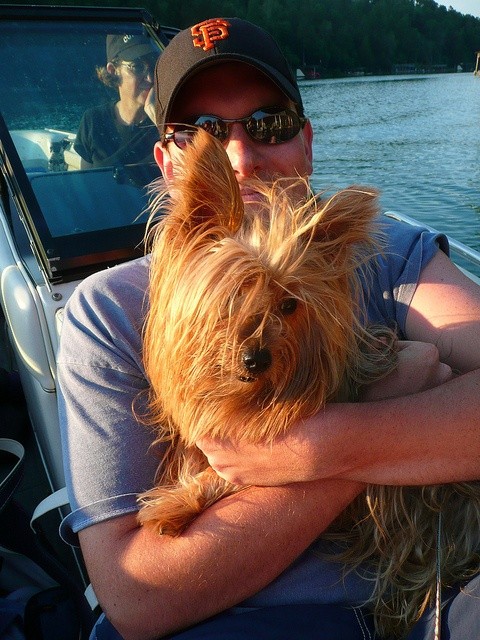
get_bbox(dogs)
[132,123,480,635]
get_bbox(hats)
[151,16,308,144]
[104,32,161,63]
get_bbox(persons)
[68,30,169,168]
[54,18,479,640]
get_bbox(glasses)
[162,103,308,147]
[120,60,157,75]
